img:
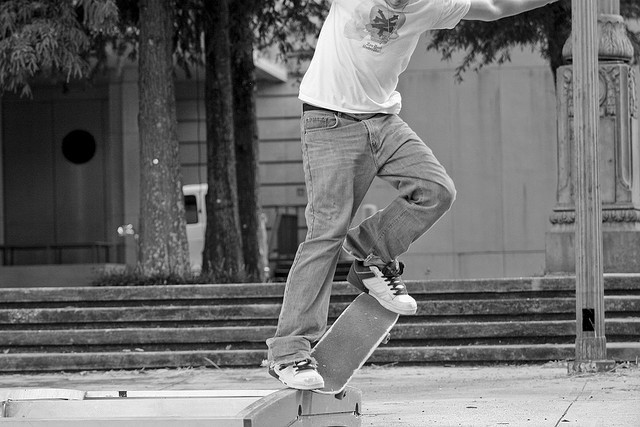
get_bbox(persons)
[268,0,561,390]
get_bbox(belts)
[301,102,377,122]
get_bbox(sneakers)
[269,358,324,390]
[347,259,417,315]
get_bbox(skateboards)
[311,291,400,399]
